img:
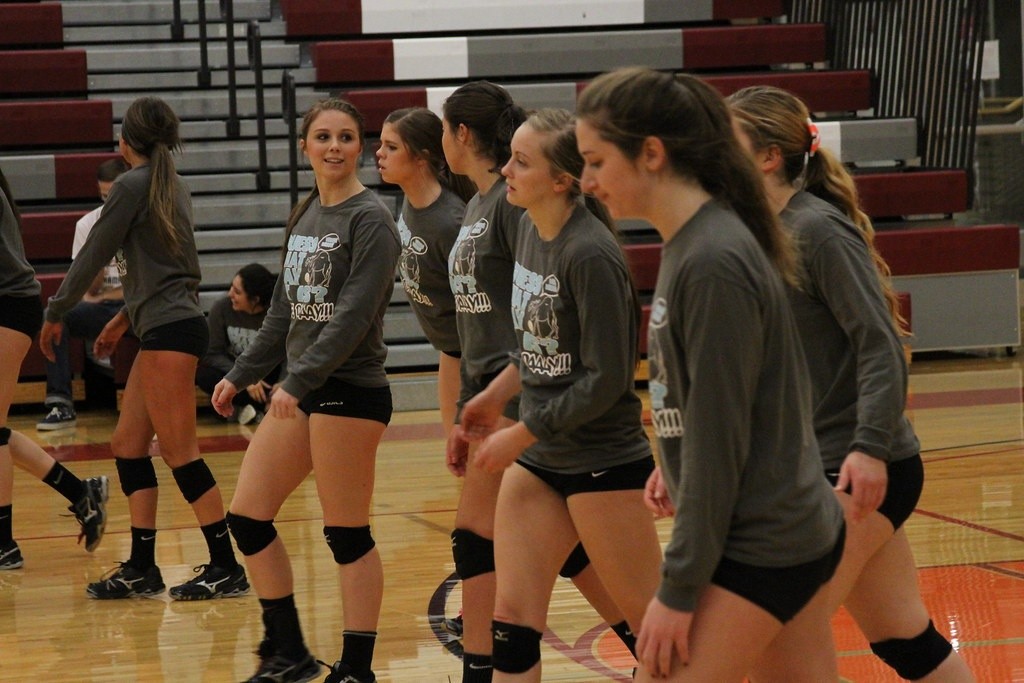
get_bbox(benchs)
[0,0,1022,385]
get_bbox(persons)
[723,86,976,683]
[376,108,476,439]
[575,67,846,683]
[0,166,109,570]
[40,97,250,601]
[207,263,289,425]
[37,158,141,431]
[211,99,403,683]
[442,81,639,683]
[461,107,663,683]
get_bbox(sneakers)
[238,404,257,427]
[236,640,323,683]
[86,561,166,600]
[0,539,24,570]
[58,474,109,553]
[316,659,377,683]
[36,395,78,431]
[168,564,251,601]
[440,606,462,638]
